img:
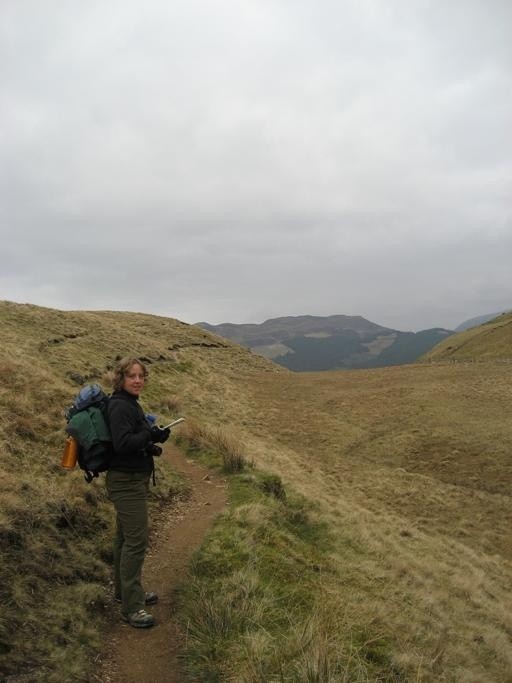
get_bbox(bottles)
[146,414,156,428]
[60,436,79,470]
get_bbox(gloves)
[152,425,171,443]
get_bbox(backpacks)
[62,384,141,483]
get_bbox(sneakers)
[120,608,153,628]
[114,591,157,605]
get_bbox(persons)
[104,355,170,628]
